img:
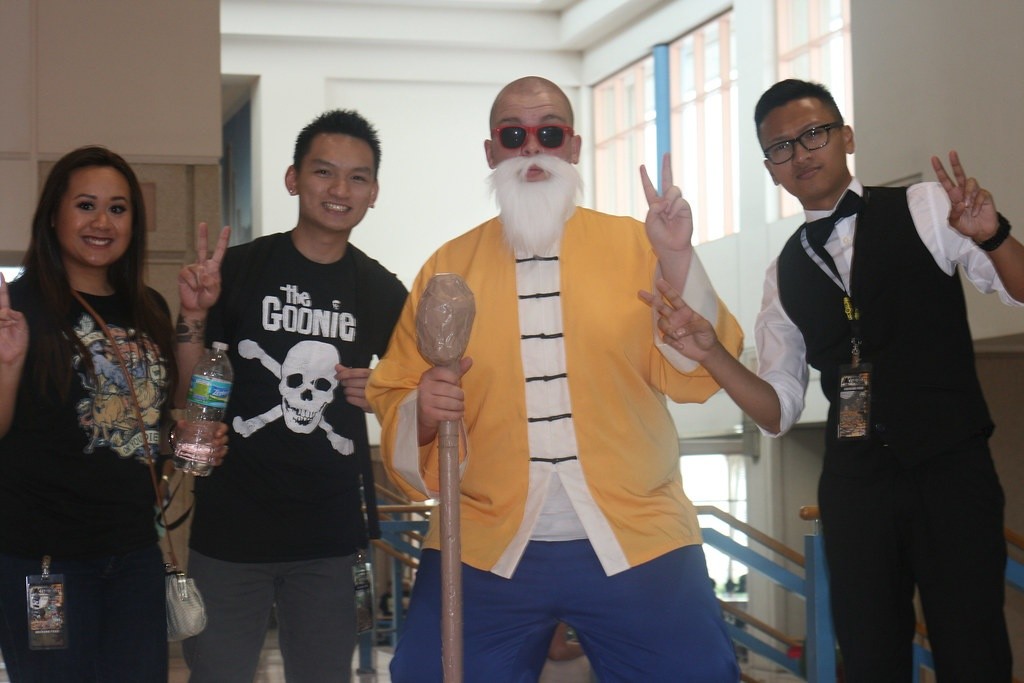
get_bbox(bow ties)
[805,189,863,246]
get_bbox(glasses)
[764,121,844,165]
[490,124,574,150]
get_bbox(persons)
[175,110,409,683]
[0,145,231,683]
[639,80,1024,683]
[366,76,746,683]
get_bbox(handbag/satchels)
[164,562,207,641]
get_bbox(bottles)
[174,340,233,474]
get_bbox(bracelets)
[160,474,174,486]
[971,212,1011,252]
[167,419,177,451]
